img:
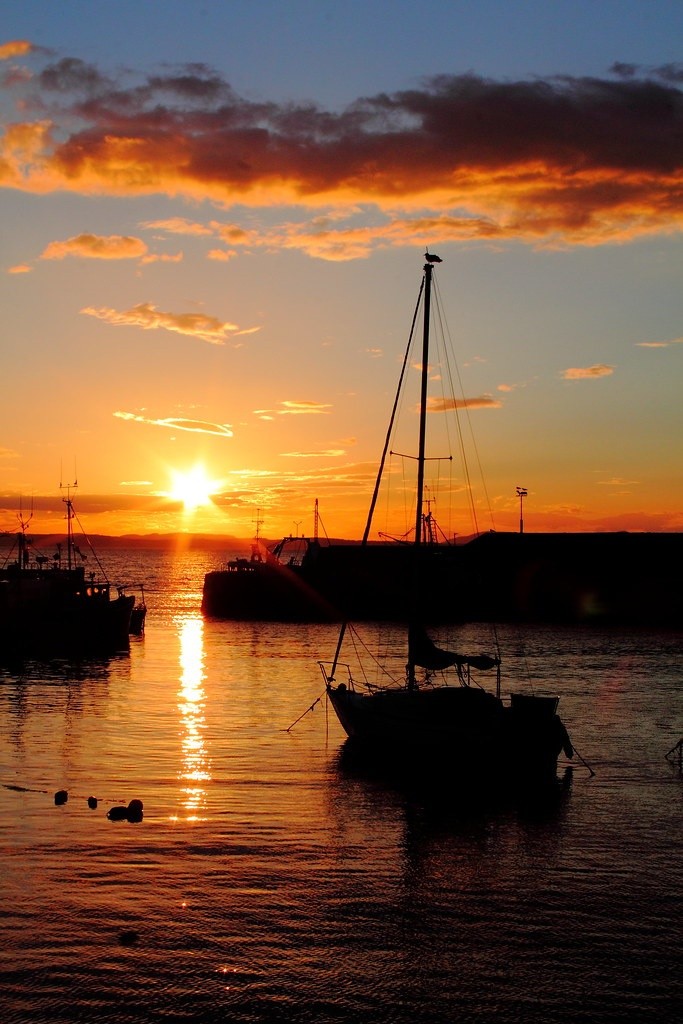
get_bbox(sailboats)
[317,243,567,764]
[0,476,148,654]
